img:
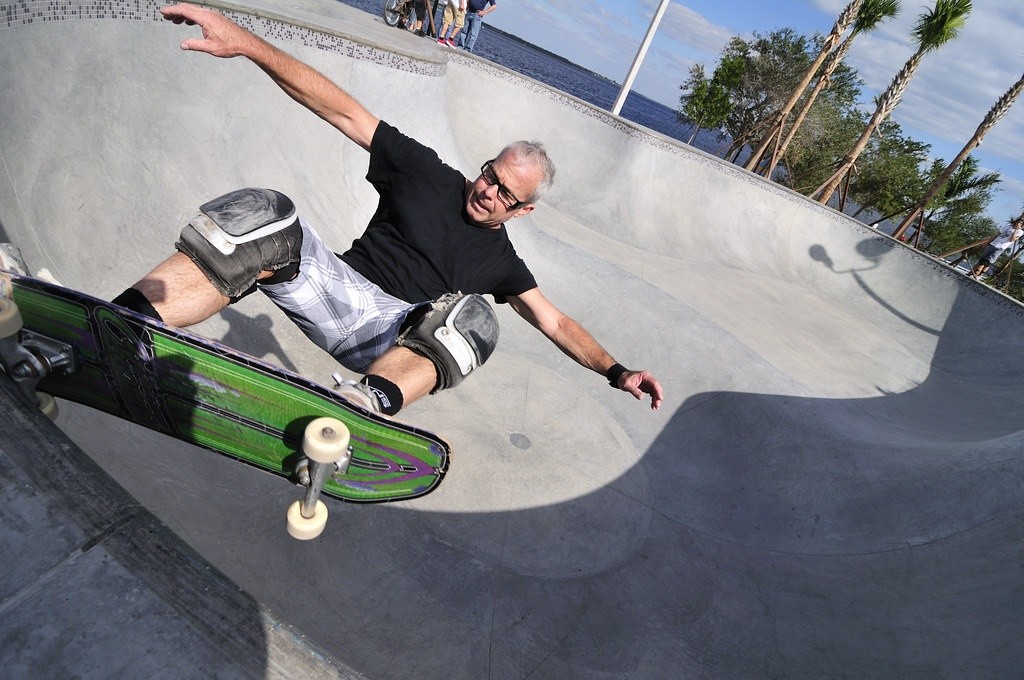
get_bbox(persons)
[396,0,497,53]
[966,218,1024,279]
[110,4,663,418]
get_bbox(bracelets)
[605,364,629,389]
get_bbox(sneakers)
[397,21,404,28]
[417,29,423,35]
[331,372,381,413]
[437,37,457,50]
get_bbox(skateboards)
[0,236,454,543]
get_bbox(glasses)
[480,158,531,210]
